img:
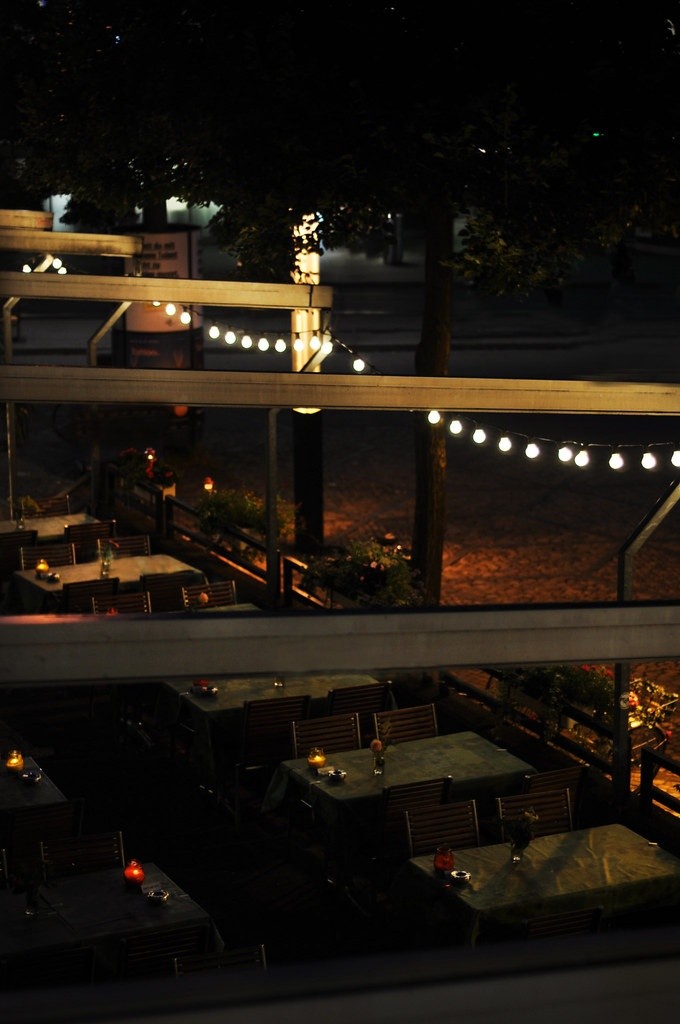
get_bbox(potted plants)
[192,484,299,555]
[486,661,613,743]
[301,537,431,613]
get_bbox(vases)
[23,893,40,920]
[117,477,176,508]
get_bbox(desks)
[405,823,680,952]
[0,754,75,842]
[261,731,538,895]
[0,862,212,966]
[162,672,380,800]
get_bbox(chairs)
[0,797,126,895]
[1,921,268,999]
[514,905,605,946]
[0,494,265,616]
[217,680,443,834]
[341,761,590,917]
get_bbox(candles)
[123,858,146,891]
[4,748,25,776]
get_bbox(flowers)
[114,446,179,486]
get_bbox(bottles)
[122,858,145,889]
[434,846,455,875]
[306,747,326,770]
[5,750,23,775]
[36,559,49,579]
